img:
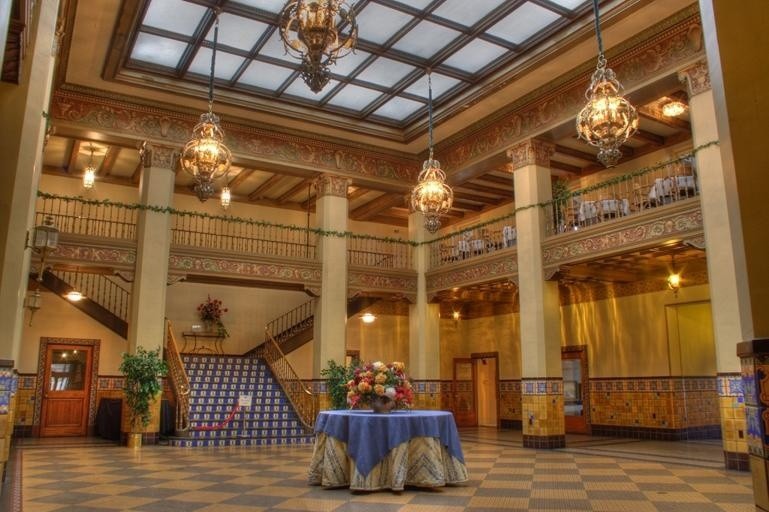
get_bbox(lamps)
[662,101,686,118]
[219,188,233,210]
[358,314,377,322]
[19,203,63,282]
[575,2,638,168]
[181,12,231,203]
[450,301,461,328]
[278,1,358,95]
[23,287,42,326]
[411,65,453,235]
[62,270,88,301]
[660,250,684,297]
[82,153,96,190]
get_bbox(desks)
[180,331,229,356]
[309,405,470,493]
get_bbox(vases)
[371,397,396,414]
[204,320,214,334]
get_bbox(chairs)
[437,174,708,266]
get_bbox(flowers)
[194,292,228,319]
[348,361,415,405]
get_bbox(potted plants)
[116,346,165,448]
